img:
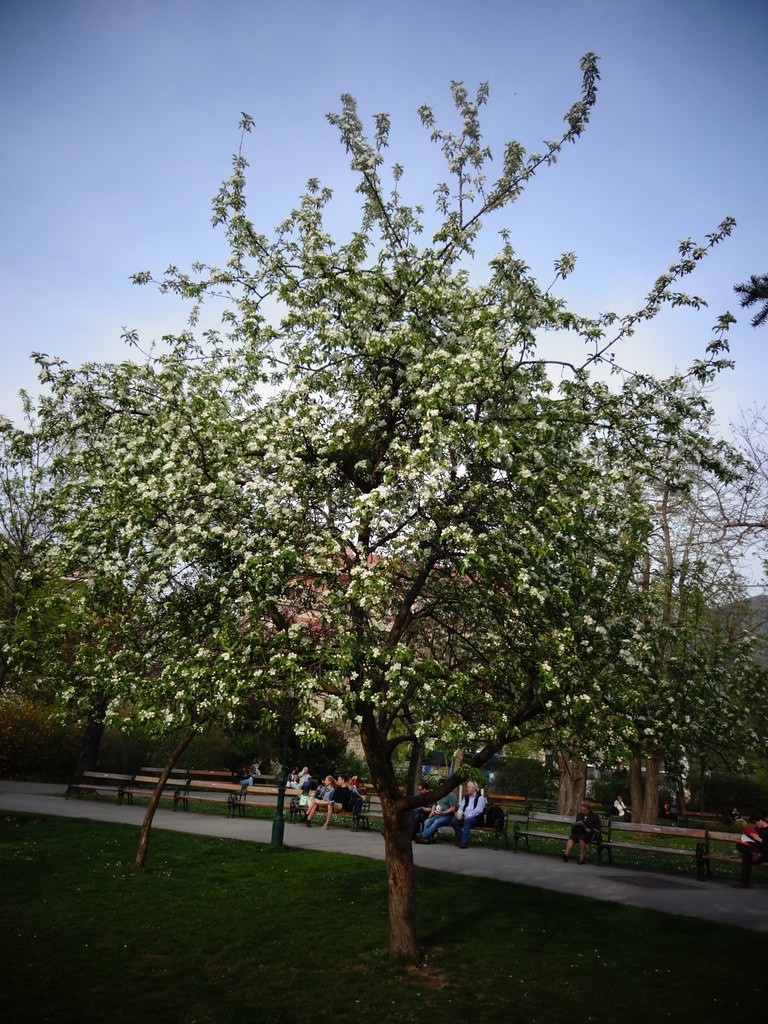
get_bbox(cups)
[435,806,441,814]
[456,810,463,820]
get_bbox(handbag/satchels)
[345,791,363,810]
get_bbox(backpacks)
[485,804,504,828]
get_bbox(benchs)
[69,766,768,881]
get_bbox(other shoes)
[559,852,568,862]
[305,820,312,827]
[459,842,467,849]
[455,838,461,846]
[300,813,308,823]
[752,851,761,863]
[578,858,585,864]
[321,825,328,829]
[731,881,749,889]
[415,833,423,840]
[416,838,432,844]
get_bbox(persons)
[614,795,631,823]
[663,801,679,827]
[730,810,768,890]
[414,778,485,849]
[300,774,350,830]
[350,776,360,795]
[730,807,741,819]
[560,801,601,864]
[288,766,312,789]
[237,763,253,786]
